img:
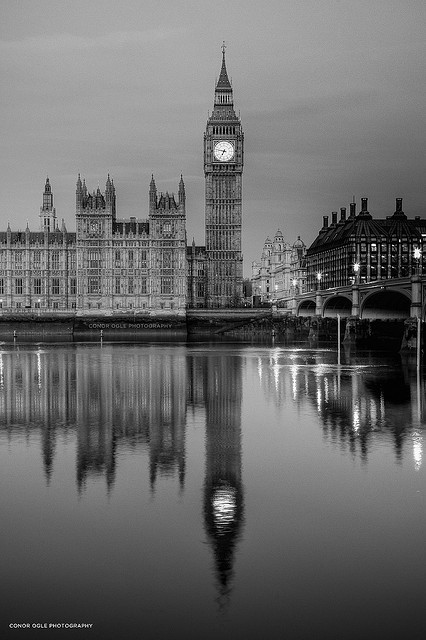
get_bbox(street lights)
[317,273,321,290]
[413,247,422,275]
[293,280,296,295]
[354,262,360,284]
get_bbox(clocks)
[213,140,236,162]
